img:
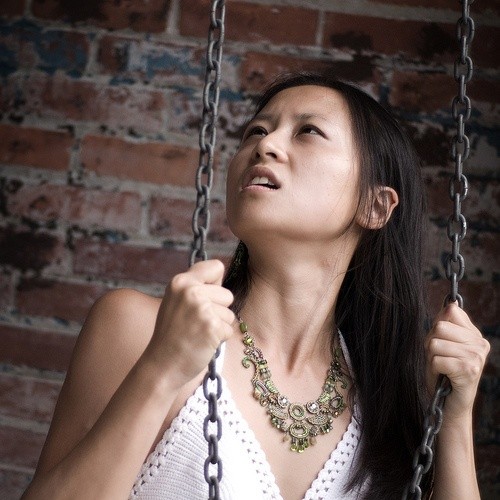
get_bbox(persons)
[16,77,493,500]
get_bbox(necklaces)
[232,302,356,453]
